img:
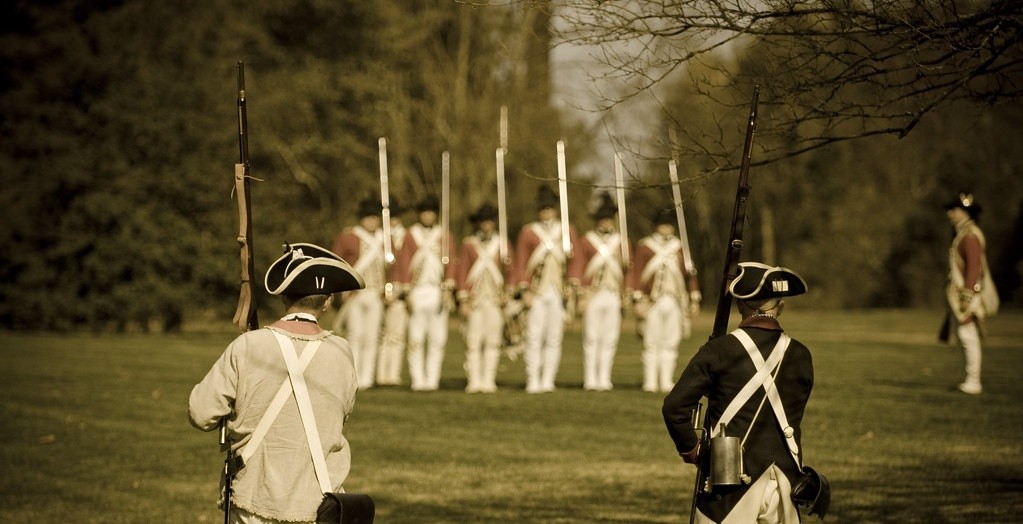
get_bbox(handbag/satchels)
[315,492,375,524]
[790,465,830,520]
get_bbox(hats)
[355,197,384,220]
[729,261,807,300]
[264,243,366,296]
[468,202,499,224]
[589,193,618,221]
[650,205,678,226]
[414,193,440,215]
[535,185,558,211]
[946,193,985,224]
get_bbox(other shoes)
[954,379,982,394]
[349,373,675,395]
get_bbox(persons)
[662,262,814,524]
[331,185,701,392]
[188,242,365,524]
[945,196,998,394]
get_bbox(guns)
[688,81,760,339]
[441,151,457,312]
[236,58,260,332]
[613,151,630,272]
[492,105,510,270]
[557,137,574,255]
[378,137,396,302]
[669,160,700,312]
